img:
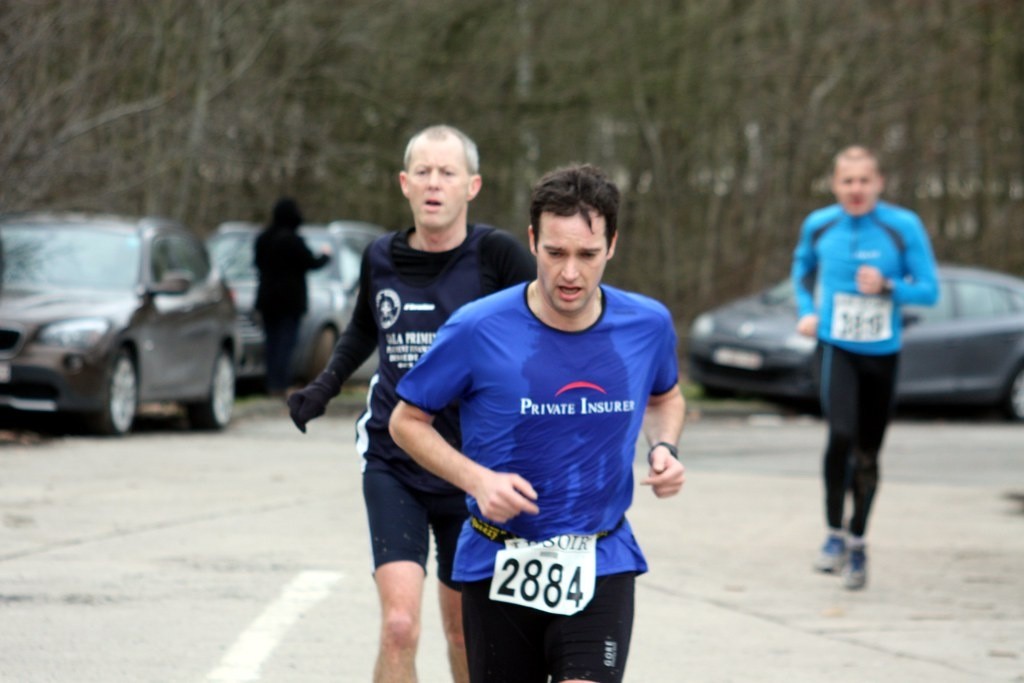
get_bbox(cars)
[688,270,1024,423]
[0,211,244,438]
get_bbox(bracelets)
[648,442,678,465]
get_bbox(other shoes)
[819,537,844,573]
[845,546,867,588]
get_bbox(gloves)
[287,373,342,433]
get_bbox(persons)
[250,199,332,397]
[388,165,685,683]
[791,146,940,588]
[288,125,536,683]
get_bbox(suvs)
[201,221,391,392]
